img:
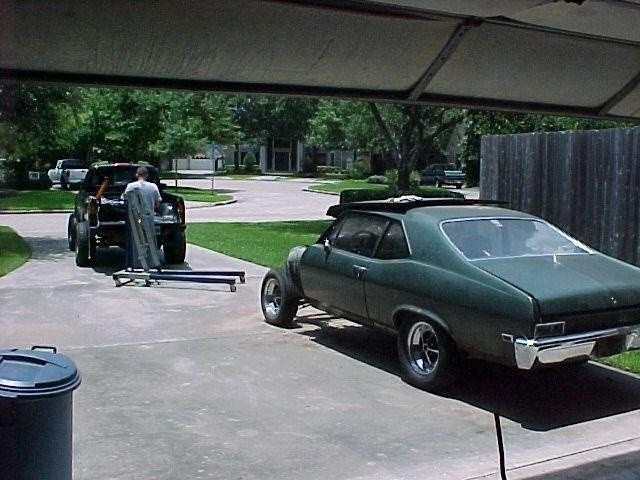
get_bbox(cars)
[260,195,640,389]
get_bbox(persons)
[120,166,166,270]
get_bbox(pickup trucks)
[48,159,89,189]
[420,163,467,189]
[67,163,186,267]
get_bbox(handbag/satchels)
[98,203,128,222]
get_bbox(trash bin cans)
[0,345,80,480]
